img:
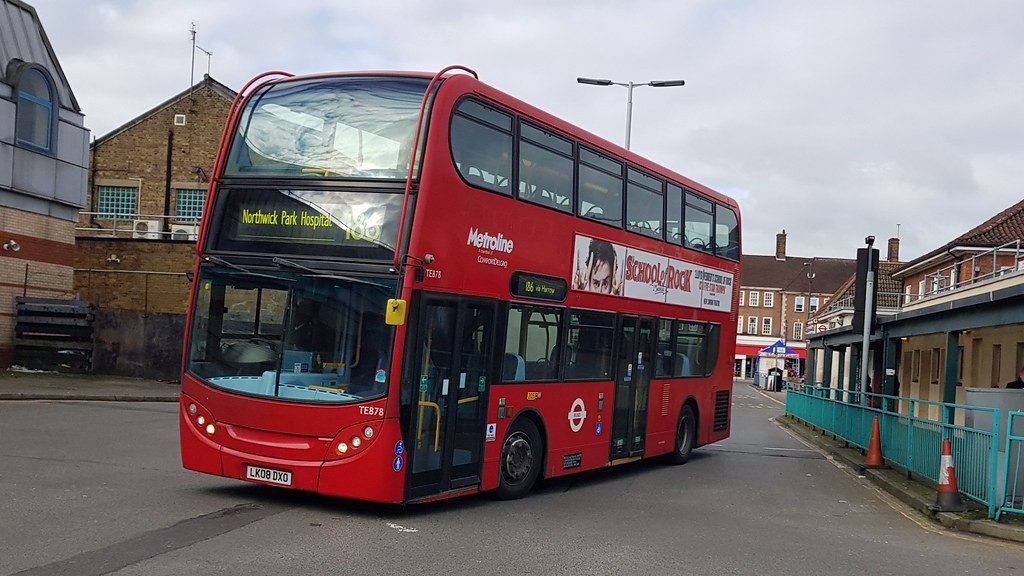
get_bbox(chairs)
[536,345,603,378]
[350,312,391,394]
[646,353,690,376]
[503,353,525,381]
[462,165,719,251]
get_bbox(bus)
[179,64,744,505]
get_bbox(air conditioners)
[132,219,159,239]
[1001,266,1015,276]
[172,225,199,240]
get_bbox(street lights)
[576,74,685,151]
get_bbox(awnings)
[735,345,806,359]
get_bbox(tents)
[757,340,800,390]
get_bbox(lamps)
[962,331,970,335]
[2,240,20,251]
[191,167,207,179]
[901,338,909,343]
[107,254,120,263]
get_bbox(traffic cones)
[859,415,891,471]
[927,438,976,513]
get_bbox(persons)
[1006,367,1024,389]
[866,374,900,412]
[787,366,797,377]
[572,237,623,296]
